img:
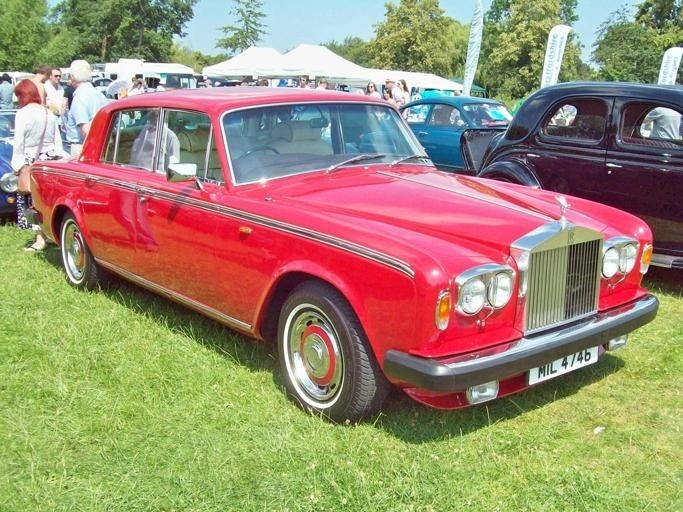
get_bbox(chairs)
[104,116,336,185]
[430,108,478,126]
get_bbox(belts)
[71,141,80,144]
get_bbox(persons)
[199,80,212,88]
[106,74,148,101]
[643,107,682,141]
[0,60,110,254]
[239,75,410,121]
[130,109,181,172]
[474,104,491,127]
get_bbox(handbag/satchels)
[17,165,32,197]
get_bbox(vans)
[138,71,203,88]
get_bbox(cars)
[28,85,659,424]
[0,111,83,216]
[460,80,683,271]
[394,94,514,170]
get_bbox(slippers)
[20,244,49,253]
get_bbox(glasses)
[54,75,61,77]
